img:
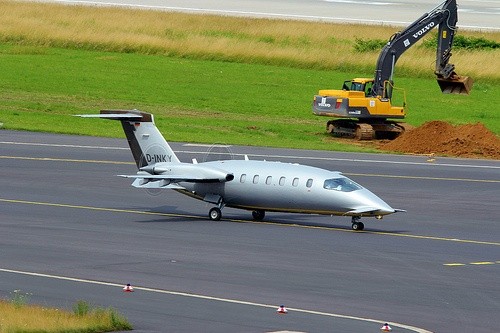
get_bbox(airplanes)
[72,108,408,231]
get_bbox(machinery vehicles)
[313,0,474,140]
